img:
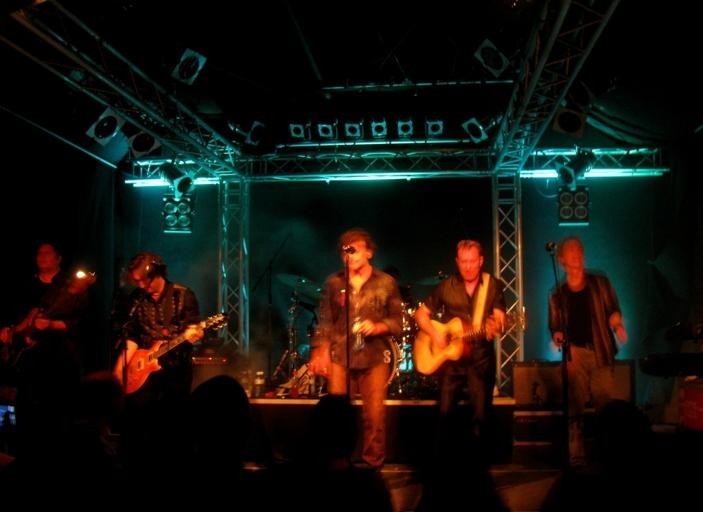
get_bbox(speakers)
[585,363,633,408]
[190,356,228,392]
[511,359,561,410]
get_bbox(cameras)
[0,403,16,427]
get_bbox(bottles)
[241,369,267,396]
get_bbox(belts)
[575,342,594,348]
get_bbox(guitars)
[119,314,228,394]
[10,271,95,363]
[411,306,526,375]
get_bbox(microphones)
[146,263,168,279]
[545,240,557,251]
[342,245,355,254]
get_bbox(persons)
[535,398,703,512]
[119,252,205,438]
[0,372,511,512]
[547,236,628,465]
[309,229,403,472]
[414,239,507,470]
[5,239,88,437]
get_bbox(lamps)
[553,143,596,195]
[458,39,517,147]
[85,43,268,199]
[284,109,452,145]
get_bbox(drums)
[393,312,413,340]
[349,334,401,397]
